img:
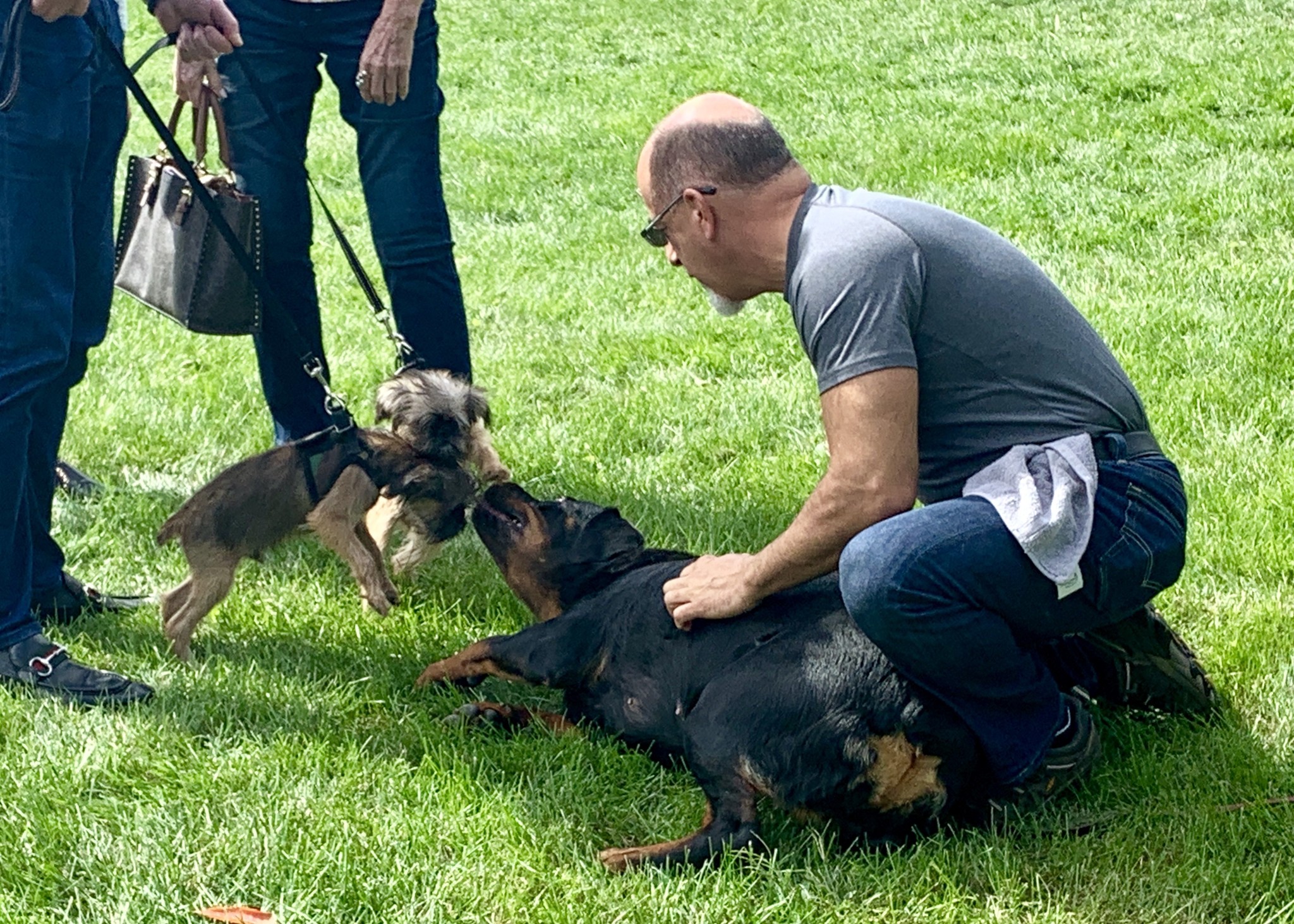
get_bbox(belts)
[1093,431,1164,462]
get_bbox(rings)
[356,71,368,88]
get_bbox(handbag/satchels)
[112,81,261,337]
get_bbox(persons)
[174,0,474,445]
[0,0,244,713]
[636,92,1225,819]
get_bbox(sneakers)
[980,694,1101,833]
[1081,607,1223,731]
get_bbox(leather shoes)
[0,633,155,712]
[33,570,161,627]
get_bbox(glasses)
[640,186,717,247]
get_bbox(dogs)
[413,479,1000,879]
[154,367,512,661]
